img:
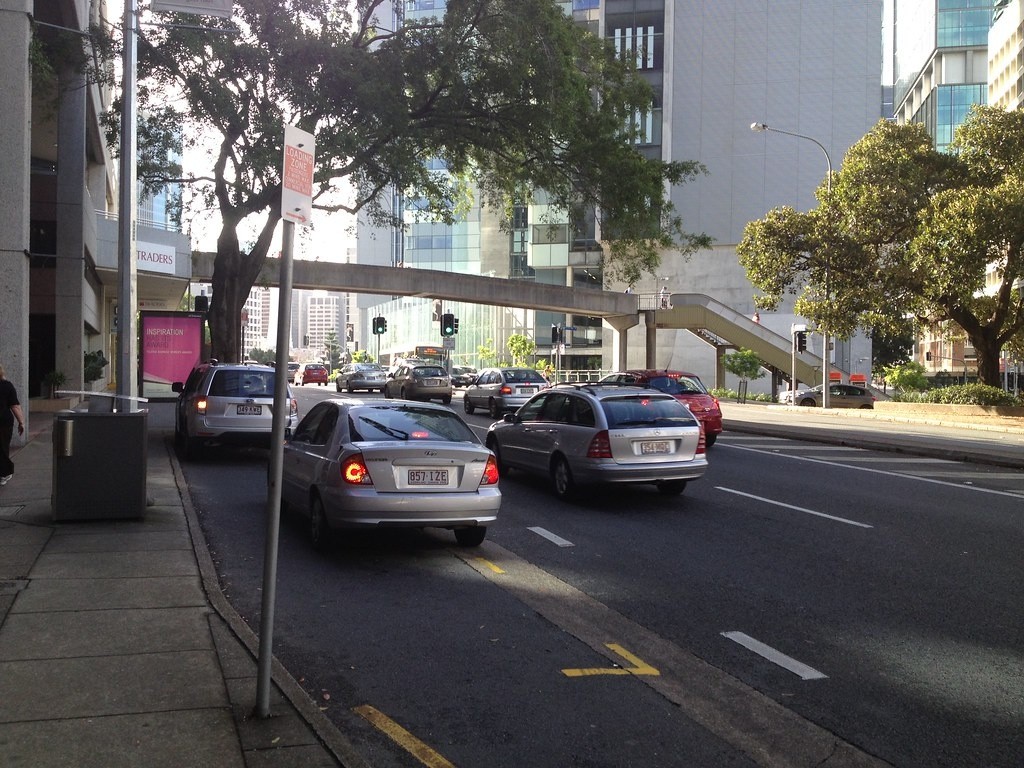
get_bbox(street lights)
[750,123,830,409]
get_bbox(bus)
[415,346,449,373]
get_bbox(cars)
[387,358,426,377]
[453,367,477,388]
[335,363,389,394]
[288,362,328,386]
[267,398,502,552]
[463,367,549,416]
[598,369,721,449]
[384,364,452,404]
[785,383,877,409]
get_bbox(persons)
[624,284,631,293]
[0,364,24,485]
[398,262,403,268]
[752,312,759,323]
[278,251,282,259]
[660,287,668,310]
[543,365,553,386]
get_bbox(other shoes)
[0,474,12,485]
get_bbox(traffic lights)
[376,317,384,334]
[384,318,387,332]
[454,319,459,334]
[443,314,453,336]
[798,332,807,351]
[195,296,208,318]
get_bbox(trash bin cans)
[51,390,148,524]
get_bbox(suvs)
[171,358,299,459]
[485,381,708,503]
